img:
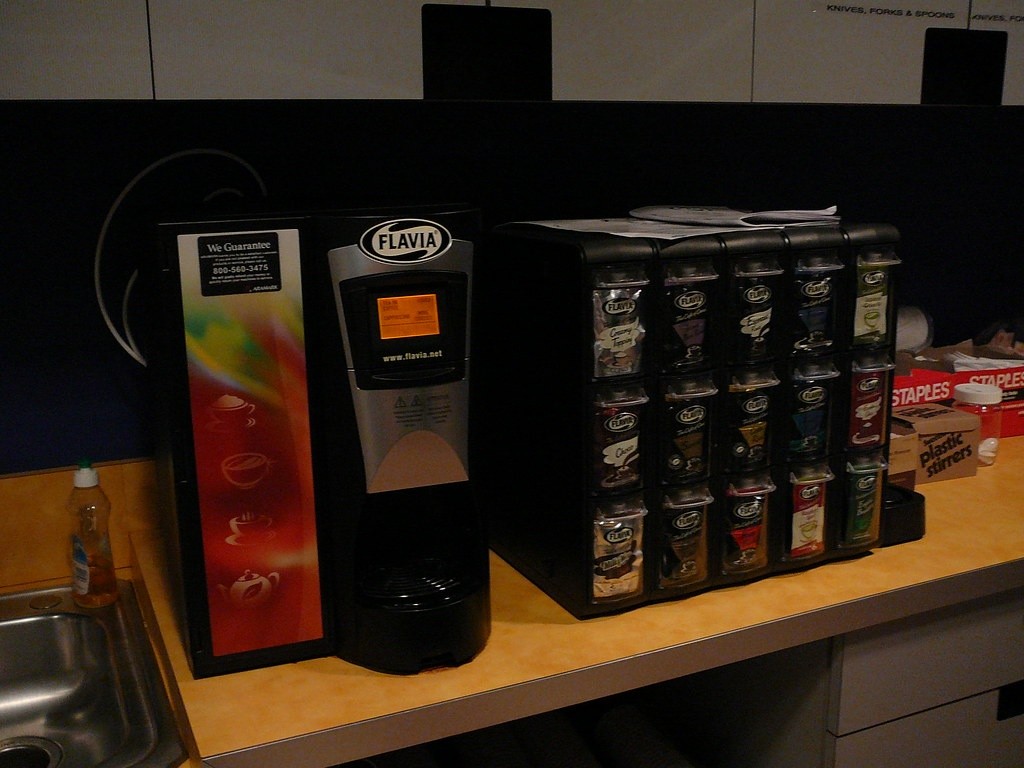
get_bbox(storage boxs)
[893,341,1024,440]
[893,404,981,484]
[160,216,333,681]
[889,416,918,492]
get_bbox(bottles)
[68,459,120,608]
[953,384,1003,467]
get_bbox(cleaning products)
[67,458,121,610]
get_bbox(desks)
[128,437,1024,768]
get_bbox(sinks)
[0,580,189,767]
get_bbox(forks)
[945,350,1008,370]
